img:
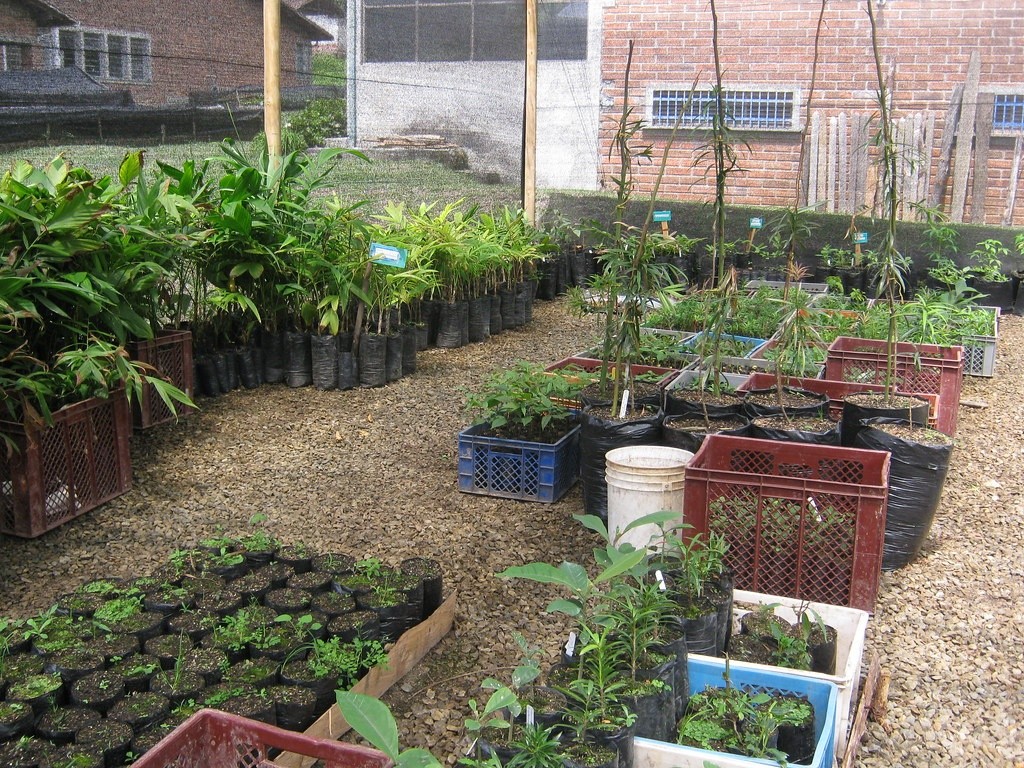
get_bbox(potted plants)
[815,246,912,300]
[930,260,966,296]
[666,297,742,420]
[0,500,857,768]
[580,261,663,410]
[961,239,1009,298]
[92,150,196,432]
[615,234,734,296]
[663,299,747,457]
[1014,280,1024,317]
[744,293,832,420]
[735,234,786,290]
[852,296,957,570]
[923,224,959,293]
[842,302,929,487]
[1011,232,1024,307]
[971,260,1013,314]
[579,298,660,531]
[191,140,601,490]
[749,292,838,502]
[0,142,130,539]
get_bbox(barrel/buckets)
[604,446,695,555]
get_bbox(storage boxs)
[637,300,797,341]
[539,355,675,388]
[0,368,131,539]
[934,318,999,378]
[681,434,891,616]
[827,334,965,437]
[959,304,1001,343]
[807,295,871,314]
[749,340,829,364]
[126,709,394,768]
[633,658,838,768]
[126,329,194,430]
[686,589,869,760]
[743,278,831,295]
[678,331,763,362]
[664,370,746,394]
[737,372,896,419]
[897,392,940,433]
[459,409,583,504]
